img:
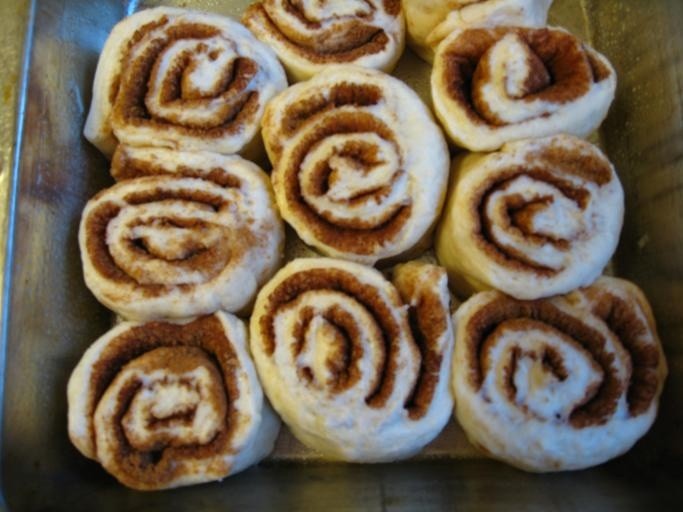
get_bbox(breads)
[77,143,286,322]
[65,309,282,493]
[83,6,289,175]
[452,276,669,475]
[248,0,406,82]
[262,66,451,265]
[250,256,454,465]
[433,134,625,301]
[404,0,551,63]
[431,21,618,151]
